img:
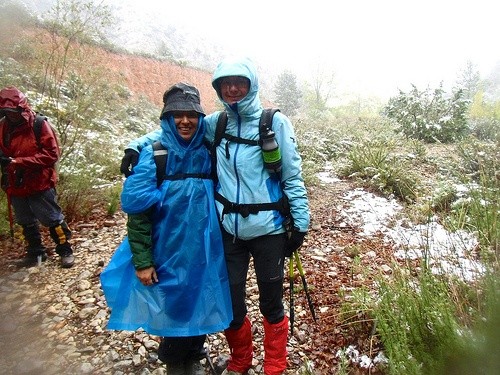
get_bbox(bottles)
[260,122,281,173]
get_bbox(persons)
[127,82,223,375]
[0,87,74,268]
[121,58,311,375]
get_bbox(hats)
[159,82,206,119]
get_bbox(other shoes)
[220,367,242,375]
[16,253,47,268]
[167,359,206,375]
[60,252,75,267]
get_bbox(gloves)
[120,149,139,178]
[284,231,308,257]
